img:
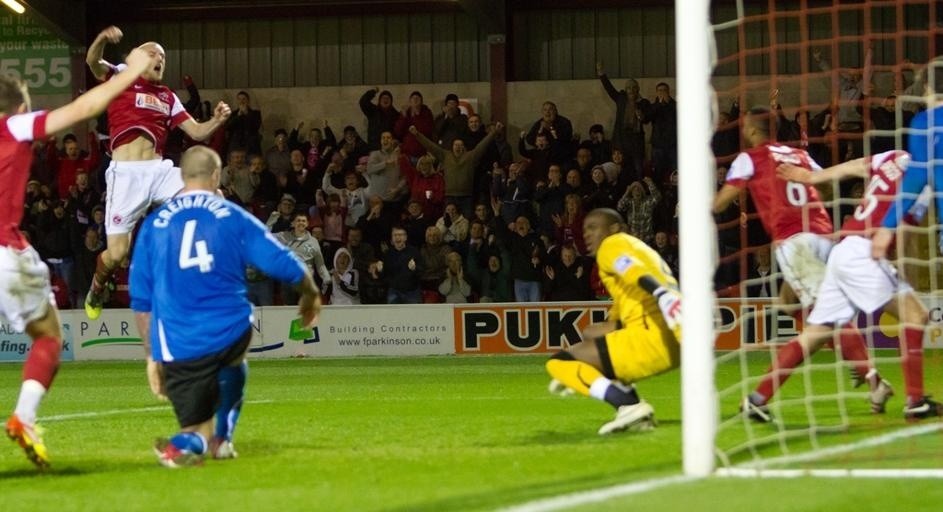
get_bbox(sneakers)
[869,373,892,414]
[208,438,236,459]
[85,275,106,319]
[153,438,201,469]
[630,420,657,432]
[739,395,773,422]
[599,399,653,435]
[7,415,51,466]
[902,400,942,420]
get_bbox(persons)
[156,58,942,304]
[713,101,894,417]
[17,80,128,311]
[128,145,324,469]
[544,208,682,435]
[84,28,234,315]
[0,45,152,468]
[738,148,943,423]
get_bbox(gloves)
[548,380,574,397]
[653,287,683,330]
